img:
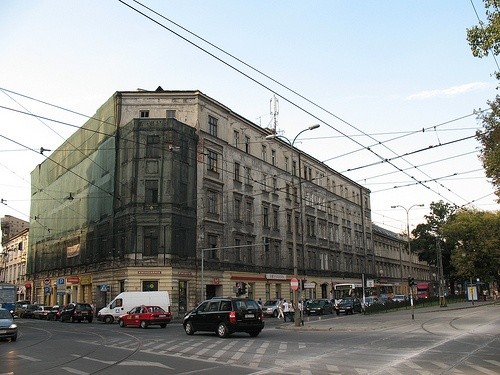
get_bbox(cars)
[335,297,363,315]
[59,302,94,323]
[0,308,18,342]
[32,306,53,320]
[0,300,33,319]
[117,304,170,329]
[361,296,384,309]
[307,299,334,316]
[46,304,67,321]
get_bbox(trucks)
[97,290,171,324]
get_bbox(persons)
[258,298,262,305]
[277,298,302,322]
[483,289,487,301]
[90,301,94,309]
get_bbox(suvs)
[182,296,265,338]
[392,295,407,303]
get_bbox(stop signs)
[290,278,299,291]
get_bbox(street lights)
[264,123,321,329]
[391,204,425,320]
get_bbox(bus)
[334,284,393,309]
[417,283,434,301]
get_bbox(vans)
[262,299,281,318]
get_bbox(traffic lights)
[241,282,248,295]
[407,277,415,287]
